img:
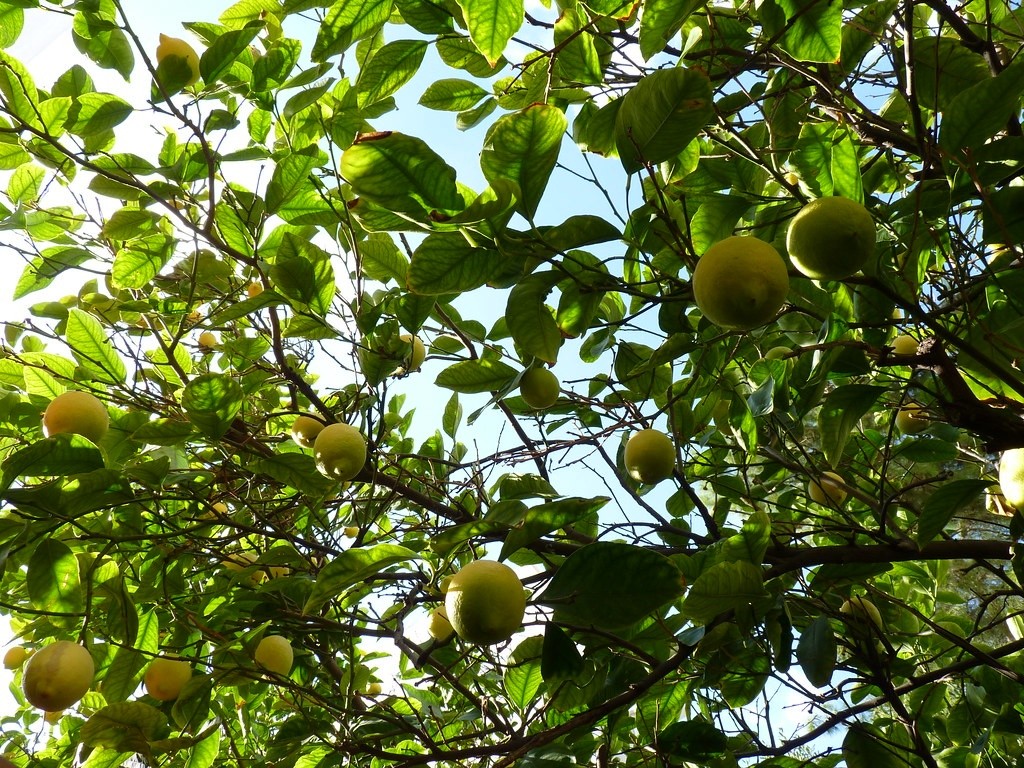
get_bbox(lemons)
[2,30,936,709]
